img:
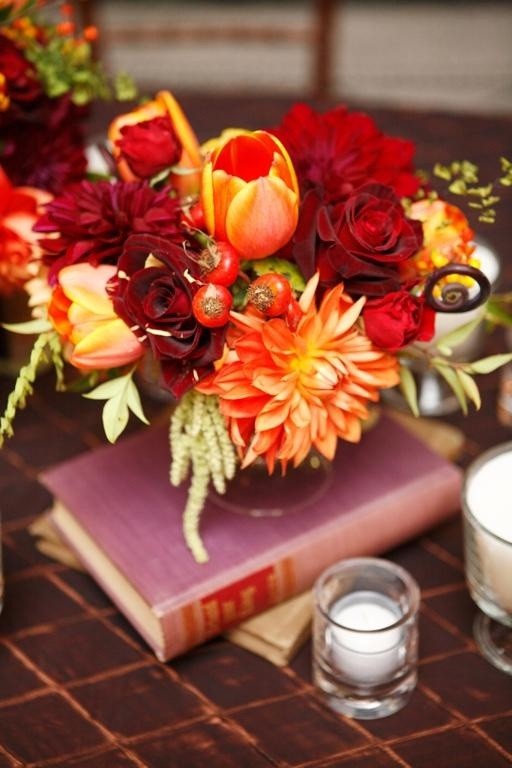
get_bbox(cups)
[458,440,512,676]
[311,555,423,722]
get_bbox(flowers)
[0,0,511,565]
